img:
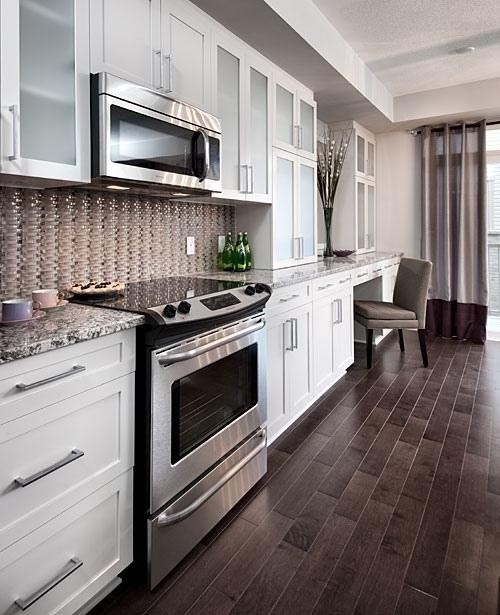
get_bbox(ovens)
[139,324,271,518]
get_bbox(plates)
[333,249,355,257]
[31,299,69,309]
[0,310,48,325]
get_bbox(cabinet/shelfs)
[90,0,211,114]
[309,271,354,396]
[0,328,136,615]
[212,30,271,204]
[272,71,317,269]
[0,0,89,184]
[356,130,377,254]
[264,281,312,429]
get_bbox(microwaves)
[89,72,224,191]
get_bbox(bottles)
[220,230,254,272]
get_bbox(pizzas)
[64,281,125,295]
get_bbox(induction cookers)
[67,274,273,328]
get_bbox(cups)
[32,288,65,307]
[1,296,43,320]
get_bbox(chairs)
[353,257,433,369]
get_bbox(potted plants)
[314,124,351,257]
